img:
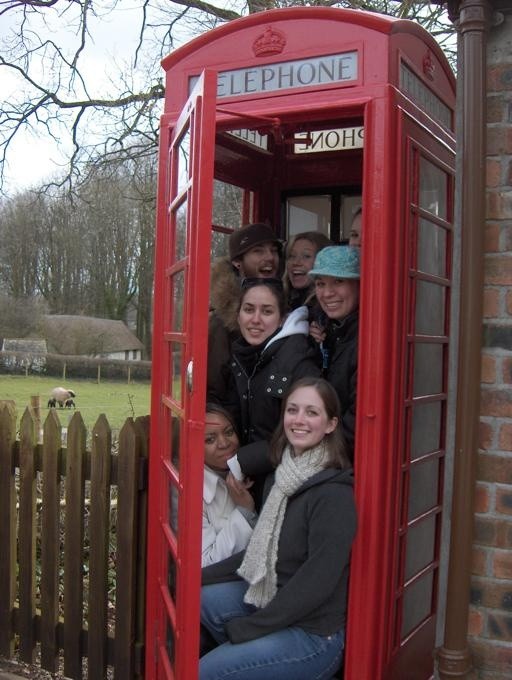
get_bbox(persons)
[167,402,259,582]
[204,208,362,507]
[196,380,359,679]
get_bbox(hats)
[308,246,360,280]
[230,223,284,261]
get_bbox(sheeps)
[48,387,76,409]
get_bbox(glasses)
[241,277,283,295]
